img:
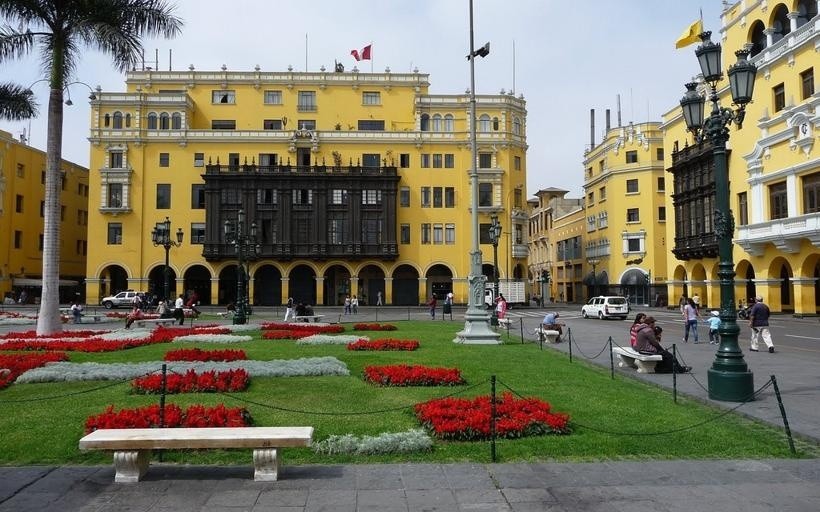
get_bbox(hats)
[754,295,764,302]
[710,309,721,316]
[643,316,656,323]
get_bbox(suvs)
[101,291,150,309]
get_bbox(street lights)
[152,216,184,304]
[679,28,767,402]
[588,259,600,295]
[489,211,503,326]
[224,206,259,323]
[233,238,261,316]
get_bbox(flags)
[675,19,702,49]
[350,44,371,62]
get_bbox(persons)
[344,294,351,315]
[284,297,314,323]
[124,292,190,330]
[377,290,383,306]
[71,301,83,323]
[351,295,358,315]
[739,300,742,310]
[528,291,542,308]
[227,302,236,315]
[542,313,563,342]
[742,299,745,310]
[748,296,774,353]
[20,290,27,305]
[495,293,506,329]
[426,293,436,320]
[626,292,722,374]
[560,292,564,302]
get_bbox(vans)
[581,295,629,321]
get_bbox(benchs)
[133,317,177,328]
[65,315,106,324]
[533,326,561,344]
[76,424,316,485]
[610,345,665,375]
[497,317,514,330]
[291,313,325,323]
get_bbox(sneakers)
[679,365,693,373]
[749,348,759,353]
[768,345,775,353]
[681,338,721,346]
